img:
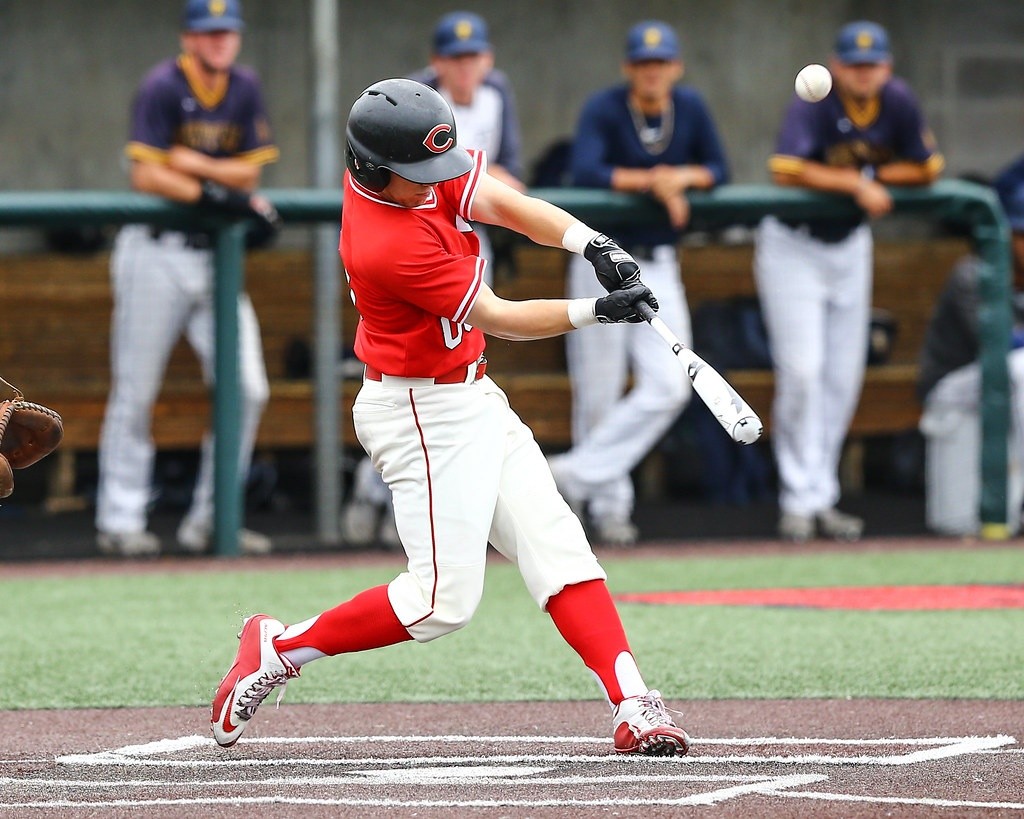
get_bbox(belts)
[780,212,858,243]
[365,356,487,385]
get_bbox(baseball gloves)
[0,398,64,498]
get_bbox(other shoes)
[177,520,274,556]
[820,509,865,538]
[97,529,160,555]
[779,508,816,541]
[343,458,383,546]
[593,515,640,545]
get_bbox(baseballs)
[795,64,834,103]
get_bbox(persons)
[406,11,524,184]
[543,18,732,544]
[95,1,281,560]
[751,22,944,545]
[208,76,694,761]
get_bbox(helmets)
[343,77,474,192]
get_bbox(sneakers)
[210,613,301,747]
[612,690,690,756]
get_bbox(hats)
[182,0,245,33]
[624,19,681,62]
[428,11,491,56]
[834,19,889,65]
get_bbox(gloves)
[584,232,640,294]
[595,286,660,324]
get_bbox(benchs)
[1,227,1024,450]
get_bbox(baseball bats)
[639,298,764,446]
